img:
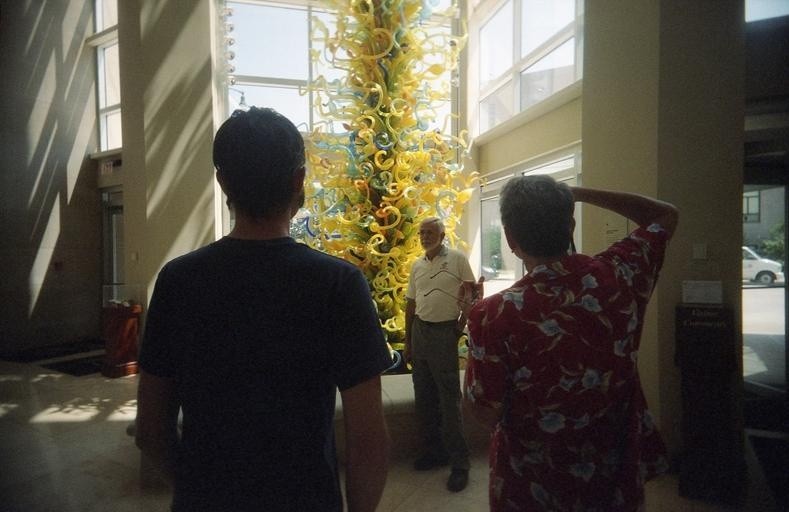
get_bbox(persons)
[133,106,392,510]
[456,174,680,510]
[405,214,476,491]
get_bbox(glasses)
[417,230,440,236]
[423,269,483,309]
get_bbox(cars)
[481,266,499,281]
[742,246,782,284]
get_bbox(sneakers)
[412,454,471,494]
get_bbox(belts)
[414,314,459,328]
